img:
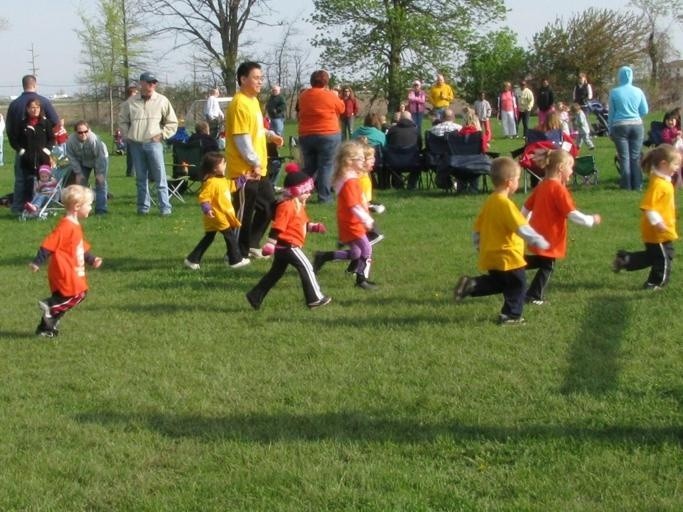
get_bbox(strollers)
[15,162,72,219]
[583,95,608,137]
[647,120,665,149]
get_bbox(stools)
[146,173,190,208]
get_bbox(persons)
[610,141,680,293]
[113,72,227,218]
[311,142,381,293]
[27,184,105,339]
[218,60,282,265]
[261,67,682,204]
[520,146,603,305]
[245,161,334,310]
[179,153,261,273]
[454,156,552,324]
[336,135,386,278]
[1,75,113,219]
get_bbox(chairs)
[370,127,599,192]
[170,140,208,187]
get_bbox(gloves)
[262,236,277,256]
[307,222,326,233]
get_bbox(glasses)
[78,130,88,134]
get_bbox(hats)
[140,72,160,82]
[38,165,52,177]
[283,163,314,197]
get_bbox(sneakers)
[184,257,200,270]
[38,299,58,337]
[455,276,472,301]
[498,314,526,325]
[614,250,629,273]
[527,295,551,306]
[308,295,332,310]
[313,251,323,273]
[224,248,268,269]
[344,269,376,289]
[643,281,662,291]
